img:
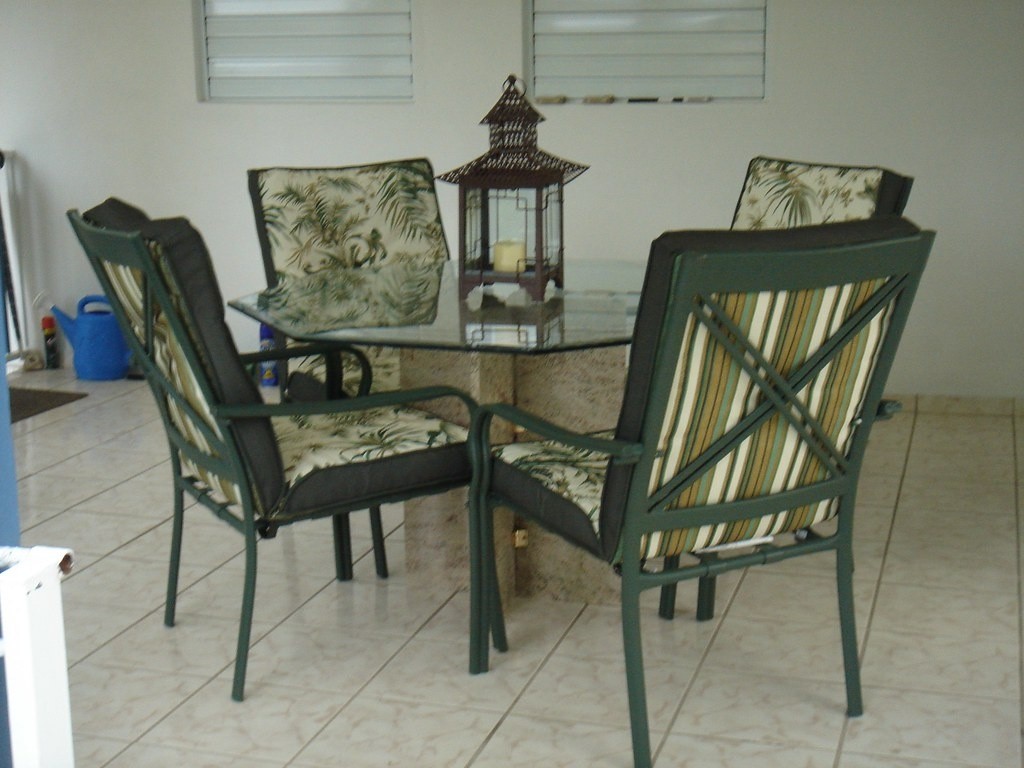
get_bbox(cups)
[494,240,526,273]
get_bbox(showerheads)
[32,286,54,310]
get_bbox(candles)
[495,240,526,272]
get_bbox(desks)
[228,260,643,614]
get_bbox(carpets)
[9,387,89,424]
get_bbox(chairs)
[248,159,449,577]
[658,158,913,621]
[66,197,510,700]
[469,213,935,768]
